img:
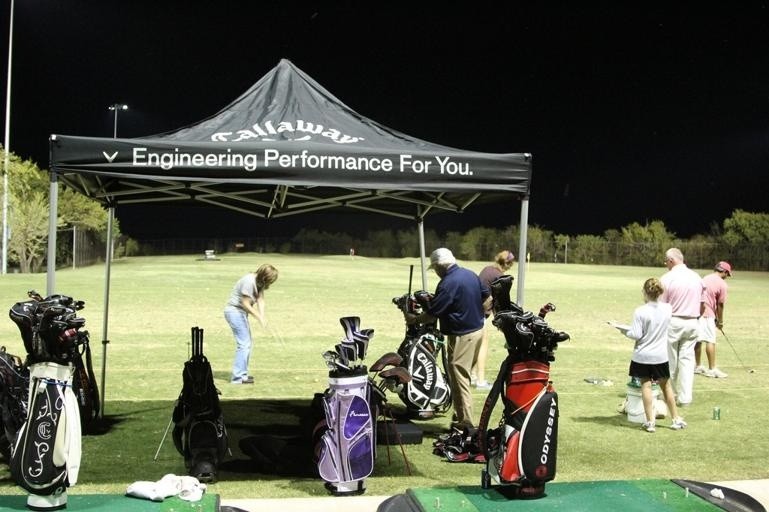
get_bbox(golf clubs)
[153,326,234,459]
[490,274,571,360]
[323,315,374,370]
[720,328,756,373]
[370,353,412,473]
[9,291,89,352]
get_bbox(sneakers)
[475,381,494,389]
[642,422,656,432]
[672,418,687,428]
[694,366,727,377]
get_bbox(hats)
[426,247,456,270]
[719,262,732,276]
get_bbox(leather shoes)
[242,376,254,383]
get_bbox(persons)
[472,250,515,391]
[223,263,279,383]
[696,261,733,378]
[619,277,689,431]
[658,248,706,405]
[414,248,492,426]
